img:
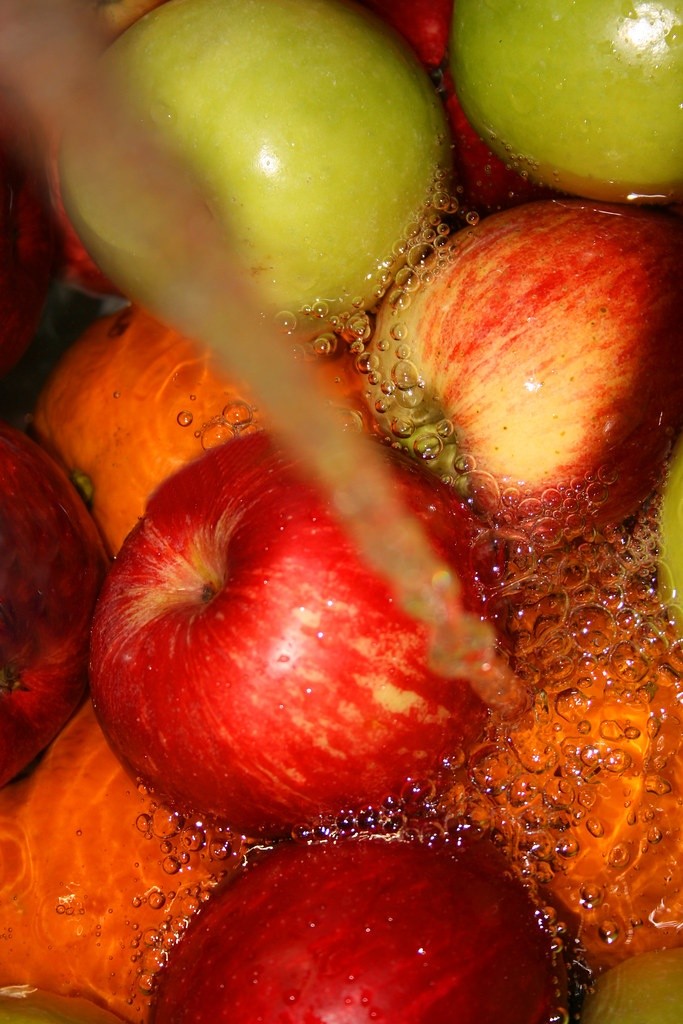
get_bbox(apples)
[0,0,683,1024]
